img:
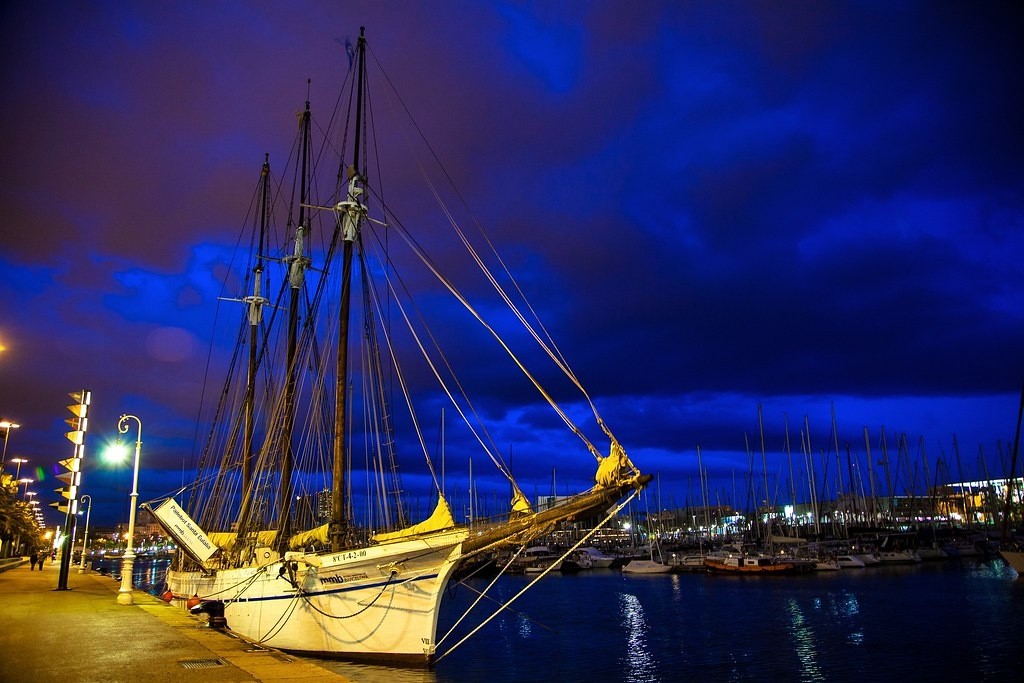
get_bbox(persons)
[29,546,59,570]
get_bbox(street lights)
[0,421,20,465]
[101,413,143,604]
[26,492,37,502]
[78,495,92,574]
[11,458,29,483]
[20,479,35,502]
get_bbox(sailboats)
[138,25,655,670]
[496,545,616,572]
[456,400,1024,574]
